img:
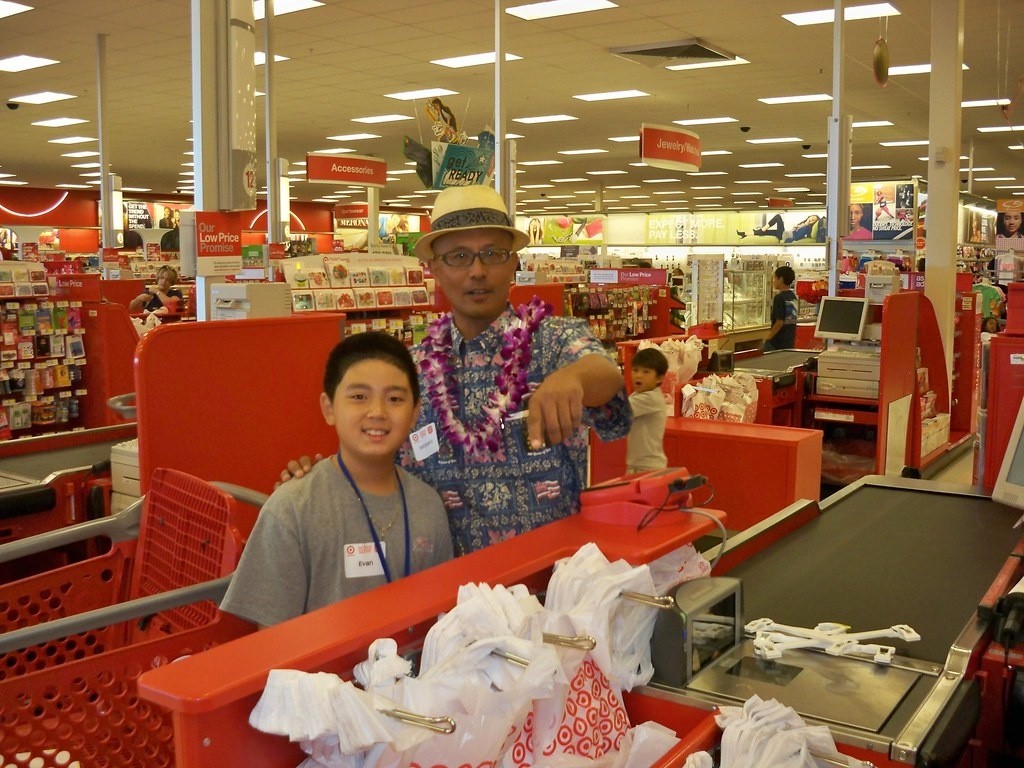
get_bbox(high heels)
[753,228,763,236]
[736,230,746,239]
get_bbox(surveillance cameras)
[7,103,19,110]
[740,126,751,132]
[541,194,545,196]
[386,203,390,205]
[802,145,811,150]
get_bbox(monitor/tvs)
[992,392,1023,510]
[814,296,868,345]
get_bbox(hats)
[414,184,531,262]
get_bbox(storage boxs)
[290,257,429,312]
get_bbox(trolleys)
[0,465,274,768]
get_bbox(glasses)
[157,265,177,274]
[432,247,516,268]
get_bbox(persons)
[626,348,668,475]
[970,212,1024,243]
[130,266,183,313]
[764,266,798,350]
[123,205,180,250]
[981,317,1001,338]
[736,185,914,244]
[273,184,635,560]
[344,214,410,250]
[218,331,454,627]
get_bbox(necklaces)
[421,294,553,452]
[337,452,411,537]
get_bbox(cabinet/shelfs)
[0,186,348,642]
[510,178,1024,767]
[277,252,452,349]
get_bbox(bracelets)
[156,290,161,296]
